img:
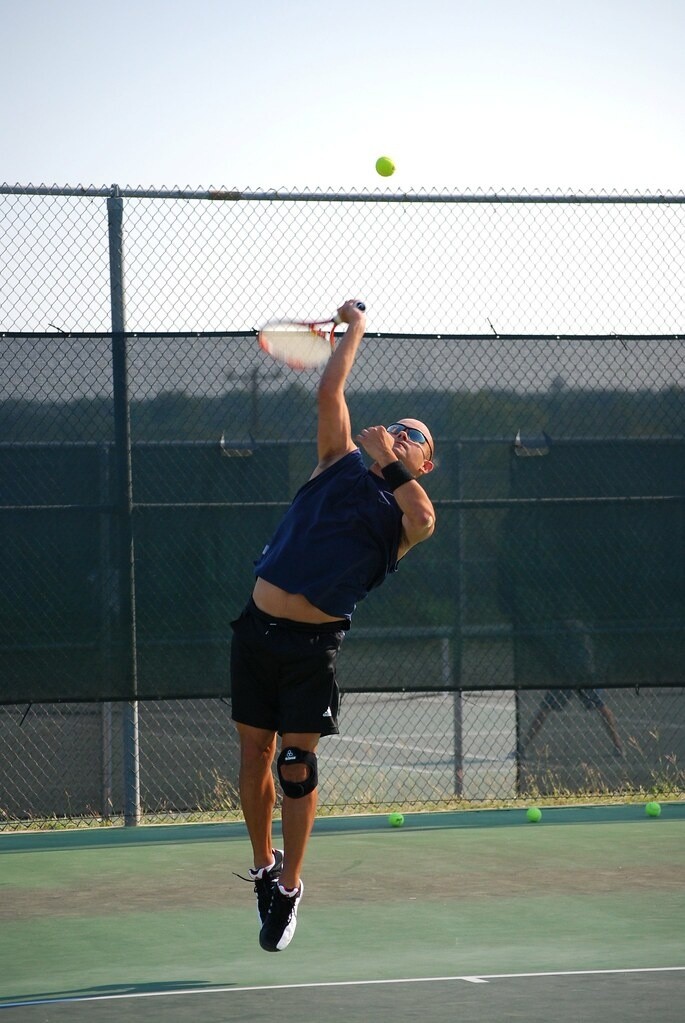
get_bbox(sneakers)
[259,879,304,952]
[232,848,284,927]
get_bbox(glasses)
[386,423,432,461]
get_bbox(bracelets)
[380,460,415,494]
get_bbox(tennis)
[375,156,396,177]
[389,812,404,827]
[645,802,661,817]
[526,808,541,822]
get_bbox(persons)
[228,298,435,952]
[507,617,625,758]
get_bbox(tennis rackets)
[257,300,367,370]
